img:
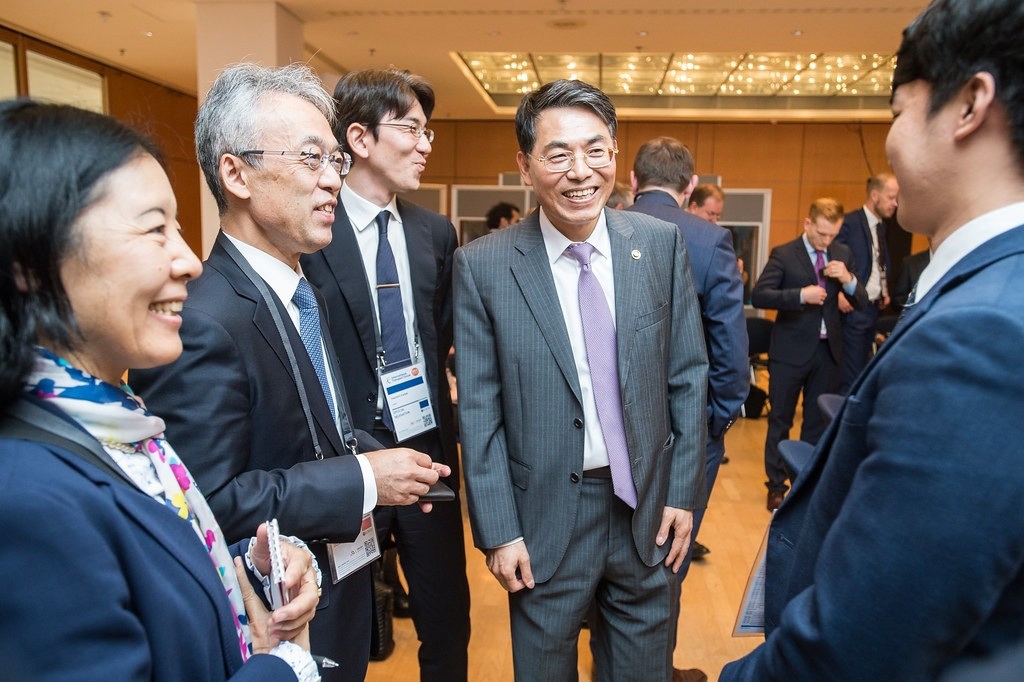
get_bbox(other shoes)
[673,667,707,682]
[767,490,784,512]
[692,541,710,556]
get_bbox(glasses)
[242,146,353,175]
[527,146,619,173]
[361,122,434,143]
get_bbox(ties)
[374,210,413,433]
[814,250,826,290]
[292,277,336,425]
[563,242,638,509]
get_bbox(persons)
[483,172,932,558]
[302,69,473,682]
[1,99,324,682]
[719,0,1024,682]
[618,136,750,682]
[451,79,710,682]
[131,62,453,682]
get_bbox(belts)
[583,465,612,479]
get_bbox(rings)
[307,581,322,597]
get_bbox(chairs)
[777,439,815,484]
[741,316,773,418]
[816,392,846,422]
